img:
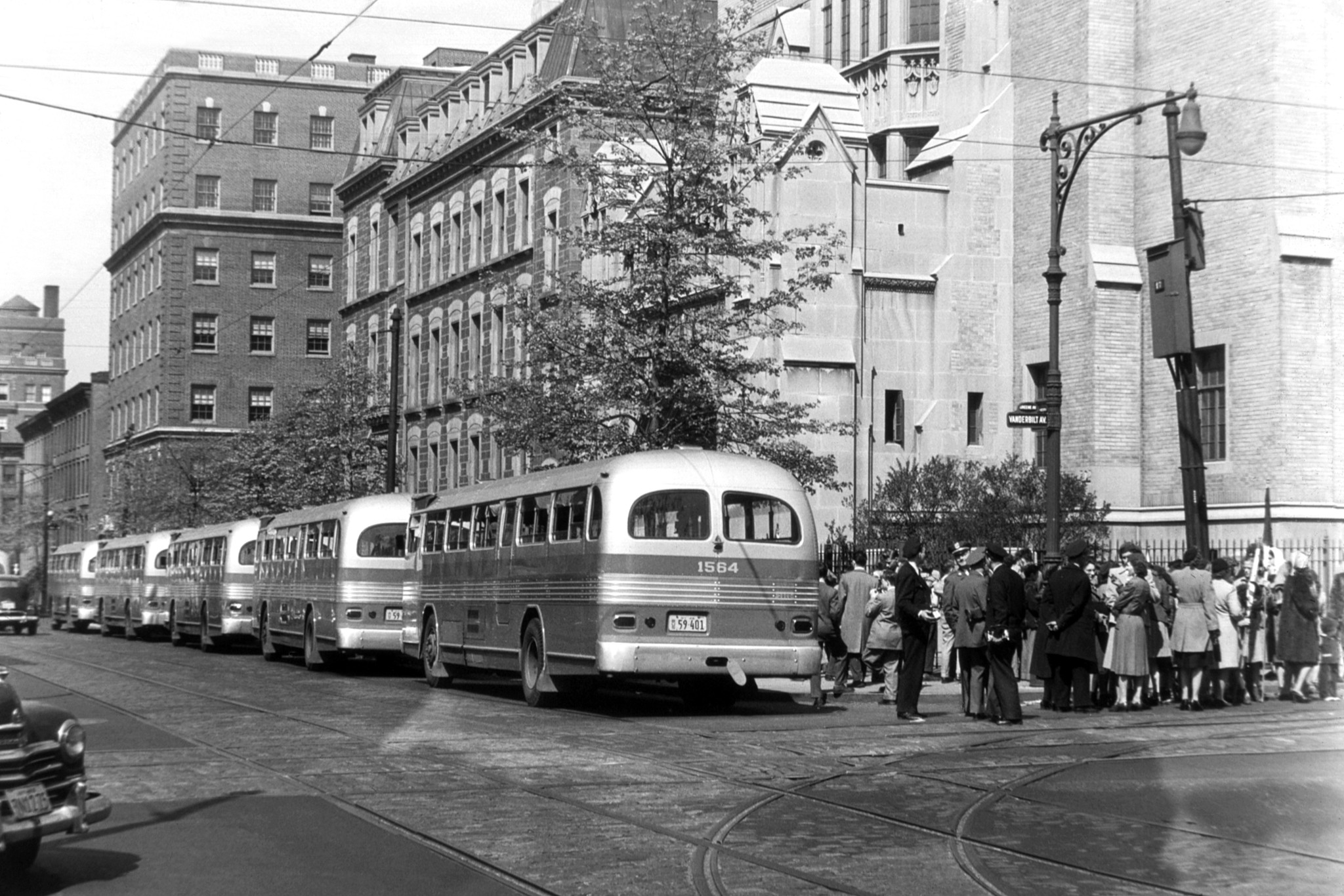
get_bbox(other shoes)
[1040,692,1343,713]
[846,679,865,688]
[941,677,954,683]
[847,675,853,680]
[965,711,974,717]
[833,683,854,694]
[877,697,897,705]
[973,713,987,719]
[996,719,1022,726]
[922,673,940,681]
[879,686,885,693]
[824,675,836,680]
[991,713,1003,723]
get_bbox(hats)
[902,541,923,559]
[1064,539,1087,561]
[982,544,1008,563]
[1212,559,1229,573]
[1183,546,1197,563]
[947,541,972,555]
[1289,552,1308,568]
[966,547,986,569]
[1321,617,1339,631]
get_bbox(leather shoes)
[897,711,927,721]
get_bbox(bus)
[46,540,110,631]
[95,530,178,640]
[166,519,260,654]
[251,493,419,670]
[401,445,823,707]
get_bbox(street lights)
[1027,81,1208,686]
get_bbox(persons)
[955,545,997,719]
[809,562,848,712]
[1168,545,1249,709]
[1009,546,1050,687]
[894,539,941,718]
[1042,539,1100,710]
[826,549,961,682]
[838,549,879,687]
[1318,617,1344,702]
[863,569,904,704]
[942,538,972,713]
[1236,541,1321,702]
[982,542,1024,726]
[1084,543,1179,708]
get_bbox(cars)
[0,574,39,636]
[0,665,112,874]
